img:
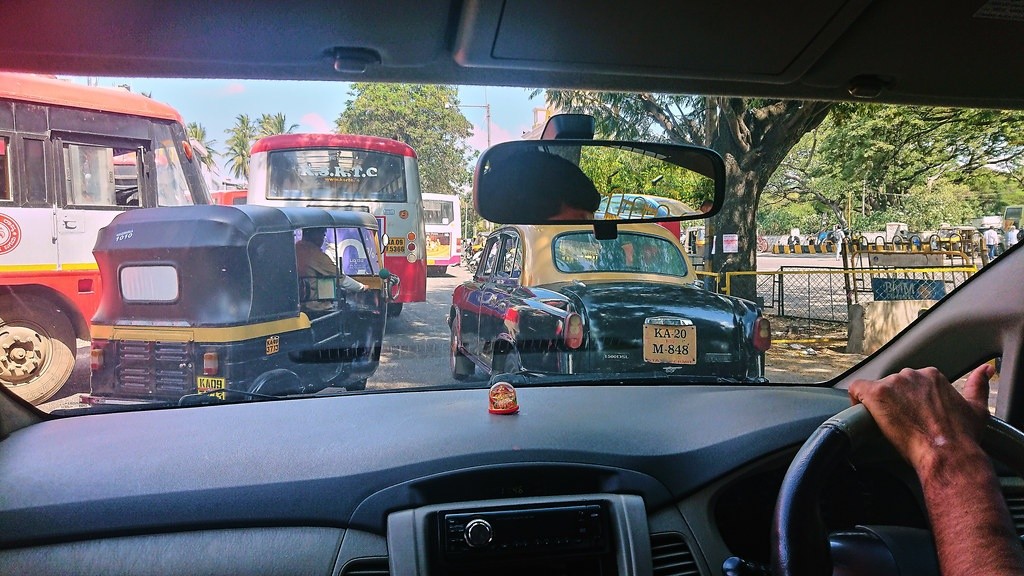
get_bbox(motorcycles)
[462,237,522,275]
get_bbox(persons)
[786,221,1019,265]
[681,230,685,251]
[848,361,1024,574]
[700,198,714,214]
[296,226,370,313]
[480,151,601,222]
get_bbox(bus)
[0,73,215,407]
[249,132,426,317]
[421,193,462,277]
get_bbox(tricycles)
[79,204,399,405]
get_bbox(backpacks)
[831,230,841,243]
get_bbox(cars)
[447,221,771,386]
[593,194,706,258]
